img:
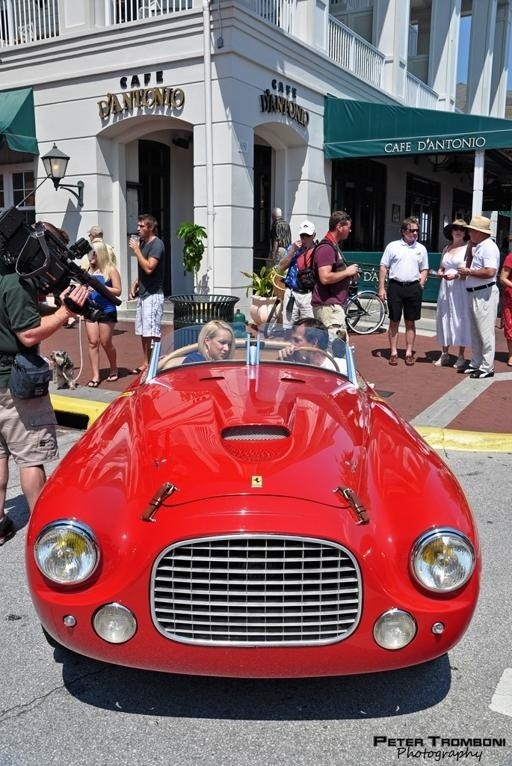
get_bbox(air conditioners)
[50,349,74,390]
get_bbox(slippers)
[298,242,337,290]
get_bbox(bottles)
[442,218,468,242]
[465,215,493,235]
[86,226,104,237]
[299,220,315,238]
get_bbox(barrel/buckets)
[64,318,78,330]
[88,362,143,387]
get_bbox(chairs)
[389,348,512,379]
[0,517,15,542]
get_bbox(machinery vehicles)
[24,322,483,680]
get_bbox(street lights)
[316,263,349,302]
[8,352,52,399]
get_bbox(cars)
[129,234,141,249]
[457,259,467,270]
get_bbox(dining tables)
[241,265,282,323]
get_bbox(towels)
[168,294,240,355]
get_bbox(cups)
[337,223,352,232]
[406,228,419,233]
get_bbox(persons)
[180,320,235,365]
[82,225,118,276]
[498,250,512,367]
[377,214,430,366]
[275,317,350,376]
[455,215,503,379]
[310,209,362,344]
[431,217,471,369]
[76,238,124,388]
[0,219,92,547]
[276,219,318,331]
[270,207,293,267]
[127,213,166,374]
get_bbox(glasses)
[419,151,448,166]
[42,140,85,207]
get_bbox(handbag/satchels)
[457,274,460,280]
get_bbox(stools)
[388,278,420,288]
[465,280,497,292]
